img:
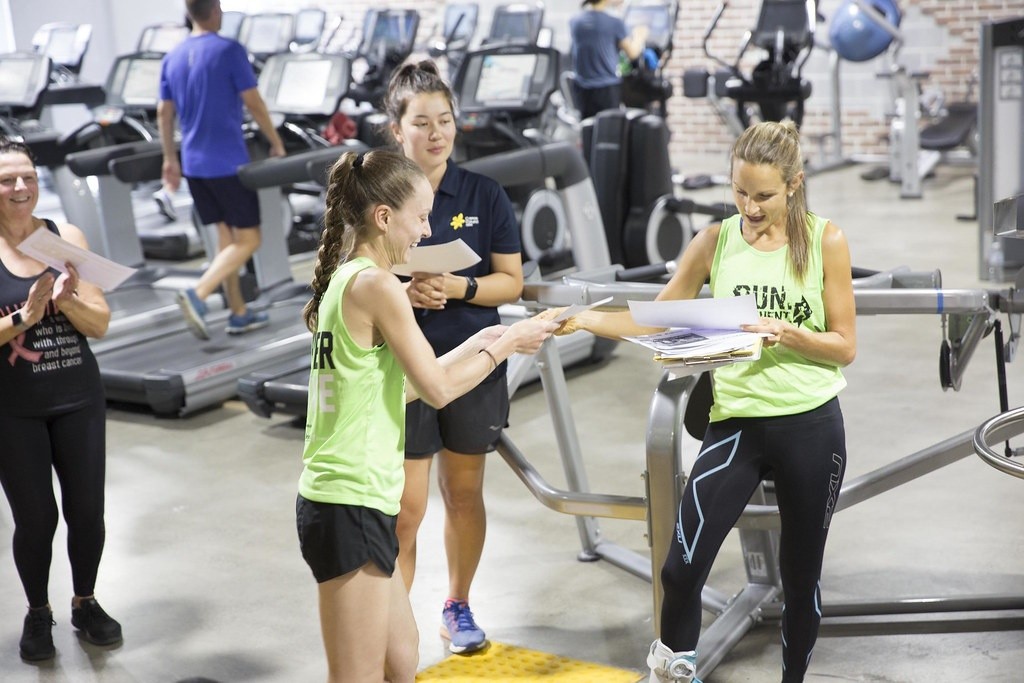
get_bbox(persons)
[570,0,648,121]
[296,151,560,683]
[535,122,857,683]
[0,141,122,660]
[157,0,285,341]
[386,61,524,651]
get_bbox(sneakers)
[440,600,486,654]
[175,288,211,340]
[71,597,122,645]
[19,608,55,664]
[225,309,268,333]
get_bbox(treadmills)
[0,1,695,433]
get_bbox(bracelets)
[479,349,498,375]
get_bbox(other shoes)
[646,639,703,683]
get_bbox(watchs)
[462,277,478,301]
[11,310,29,331]
